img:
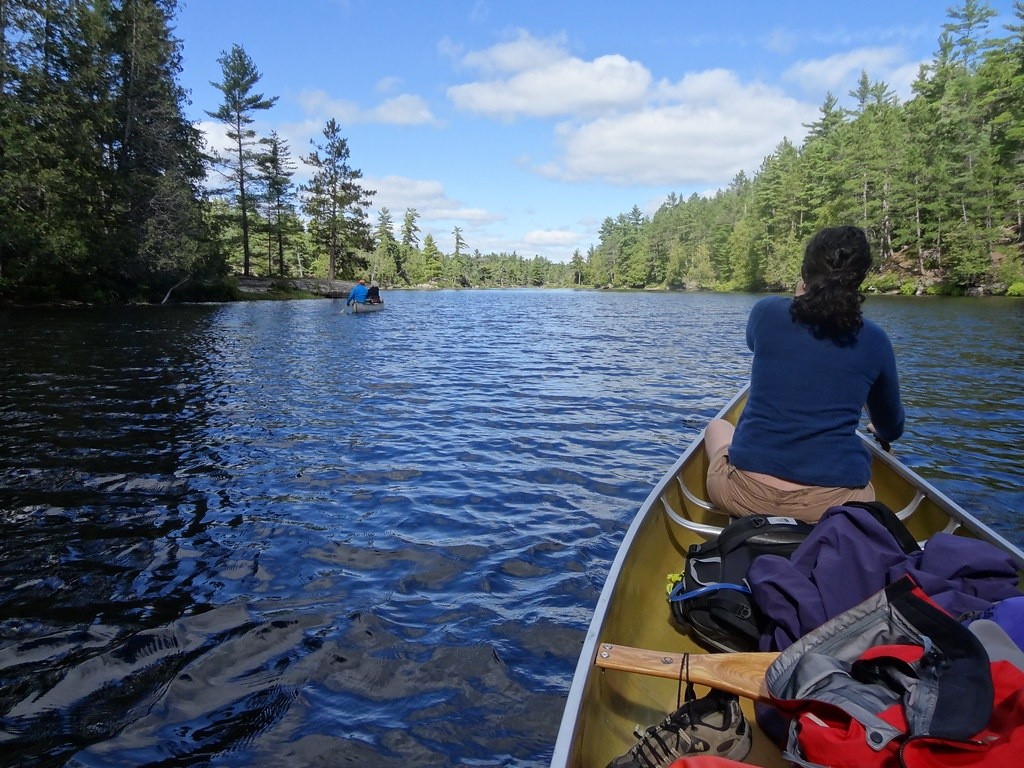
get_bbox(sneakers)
[604,690,752,767]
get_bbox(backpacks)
[668,514,815,655]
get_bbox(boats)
[352,298,385,314]
[552,374,1022,766]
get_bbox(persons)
[693,218,908,529]
[346,278,368,307]
[366,280,381,303]
